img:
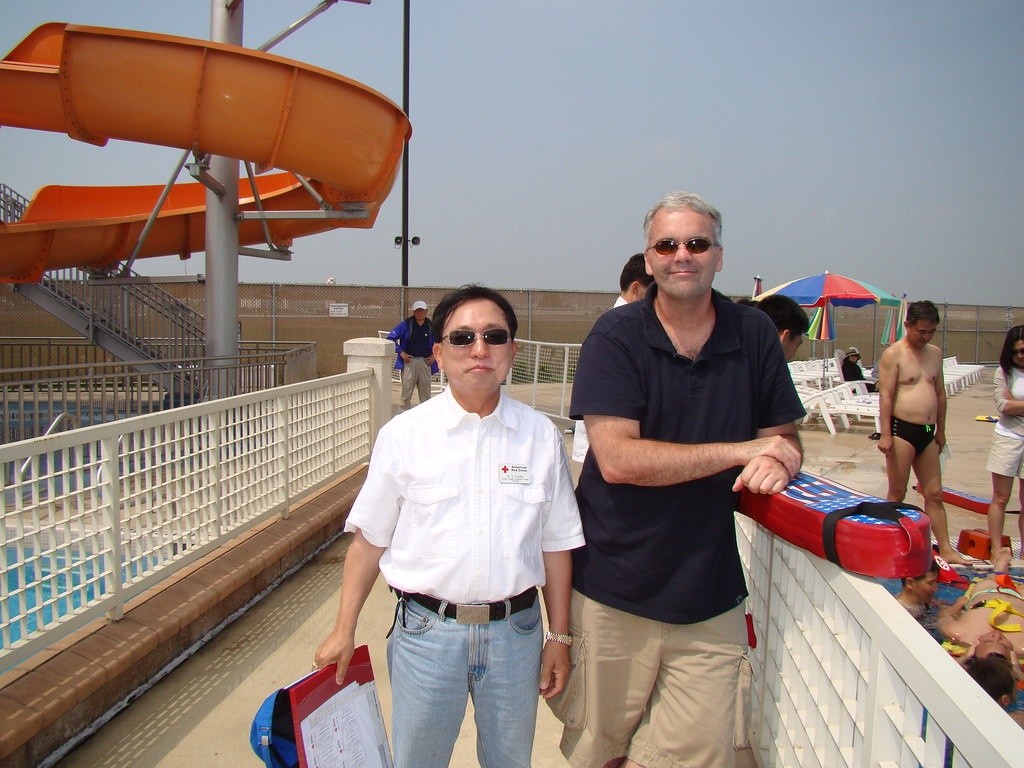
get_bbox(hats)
[411,301,427,313]
[844,347,862,363]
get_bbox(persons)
[895,559,941,619]
[386,300,439,413]
[842,346,879,393]
[311,282,570,768]
[877,300,972,565]
[539,193,807,768]
[936,547,1024,661]
[960,655,1024,731]
[986,324,1024,566]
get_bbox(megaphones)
[412,237,420,245]
[394,236,402,245]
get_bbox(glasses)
[851,354,859,357]
[651,239,720,254]
[439,329,513,346]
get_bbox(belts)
[409,587,538,625]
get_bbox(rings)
[312,658,319,668]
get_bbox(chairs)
[787,349,986,434]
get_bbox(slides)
[0,21,412,286]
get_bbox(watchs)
[546,631,573,647]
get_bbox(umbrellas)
[752,271,902,390]
[881,291,907,346]
